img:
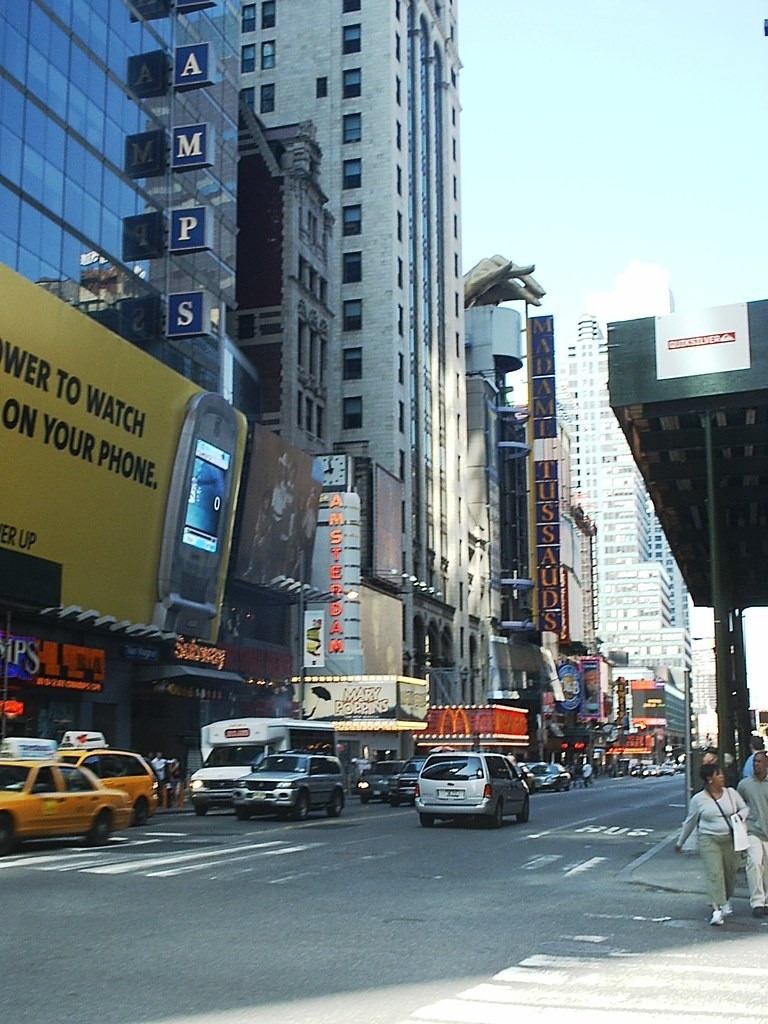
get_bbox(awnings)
[137,664,250,685]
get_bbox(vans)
[414,751,532,828]
[187,717,338,815]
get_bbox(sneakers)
[720,900,733,916]
[710,909,724,926]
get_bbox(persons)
[242,454,321,585]
[302,708,316,720]
[737,751,768,917]
[741,736,768,779]
[675,763,751,925]
[565,760,594,788]
[146,749,183,806]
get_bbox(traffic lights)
[562,739,570,749]
[573,740,587,749]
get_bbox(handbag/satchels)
[731,814,751,851]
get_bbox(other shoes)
[753,905,768,918]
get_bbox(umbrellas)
[311,686,332,707]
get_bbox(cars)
[358,760,403,803]
[56,732,162,827]
[0,738,134,856]
[510,759,572,797]
[393,753,431,808]
[630,763,679,778]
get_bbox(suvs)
[231,752,346,821]
